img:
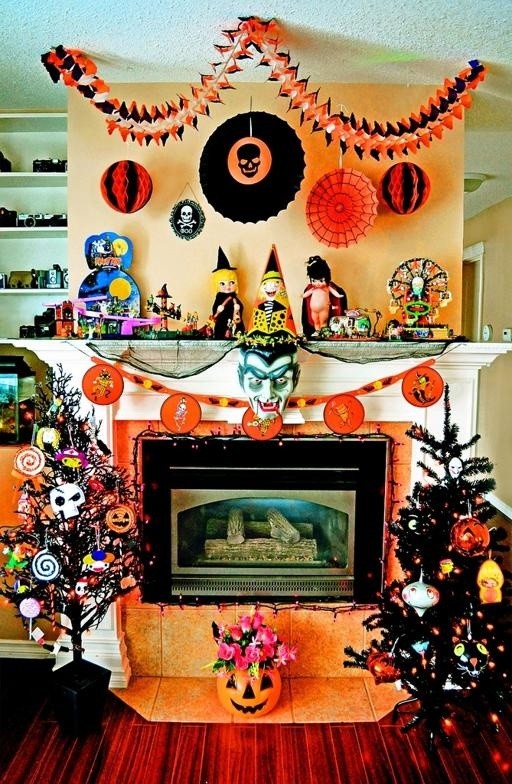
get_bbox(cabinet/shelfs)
[0,113,68,339]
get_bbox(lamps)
[464,173,487,195]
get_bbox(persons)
[299,255,350,338]
[206,245,246,340]
[246,243,299,339]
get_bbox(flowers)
[200,612,301,680]
